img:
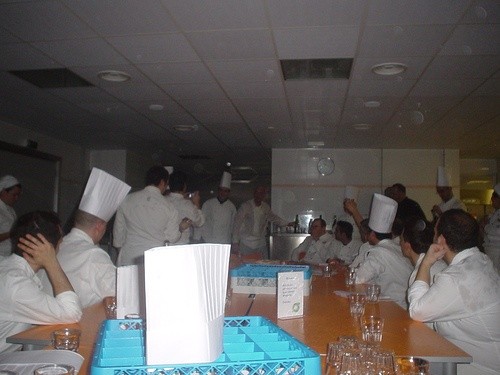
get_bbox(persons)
[398,218,449,332]
[165,172,204,245]
[291,218,338,264]
[408,208,500,375]
[330,220,363,266]
[387,182,429,232]
[477,183,500,273]
[232,180,273,261]
[113,167,193,267]
[431,164,467,220]
[0,212,82,375]
[38,168,131,310]
[0,176,26,237]
[344,193,414,311]
[194,172,237,244]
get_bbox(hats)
[368,193,398,234]
[219,170,232,190]
[436,166,453,187]
[0,175,22,191]
[78,167,132,222]
[494,183,500,196]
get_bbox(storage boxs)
[231,263,313,296]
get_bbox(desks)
[7,257,473,375]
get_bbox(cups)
[365,283,380,305]
[103,296,118,318]
[345,268,356,287]
[349,293,365,318]
[323,264,330,277]
[53,328,81,352]
[225,288,232,307]
[35,363,74,375]
[360,315,384,343]
[326,336,429,375]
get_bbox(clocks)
[317,158,336,176]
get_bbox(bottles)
[332,215,337,228]
[309,219,313,227]
[320,215,322,218]
[294,214,299,233]
